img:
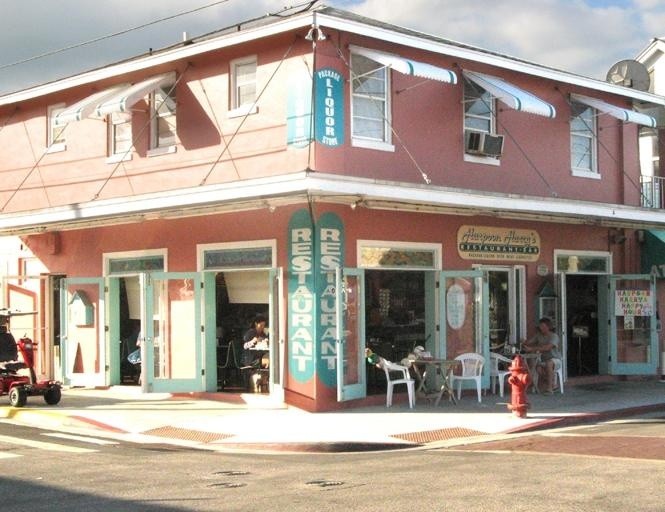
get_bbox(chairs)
[118,336,269,392]
[357,344,567,409]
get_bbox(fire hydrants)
[506,352,536,420]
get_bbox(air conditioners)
[464,128,504,158]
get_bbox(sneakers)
[542,384,559,396]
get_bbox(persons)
[366,308,387,335]
[242,316,268,369]
[520,318,562,397]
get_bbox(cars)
[365,306,419,347]
